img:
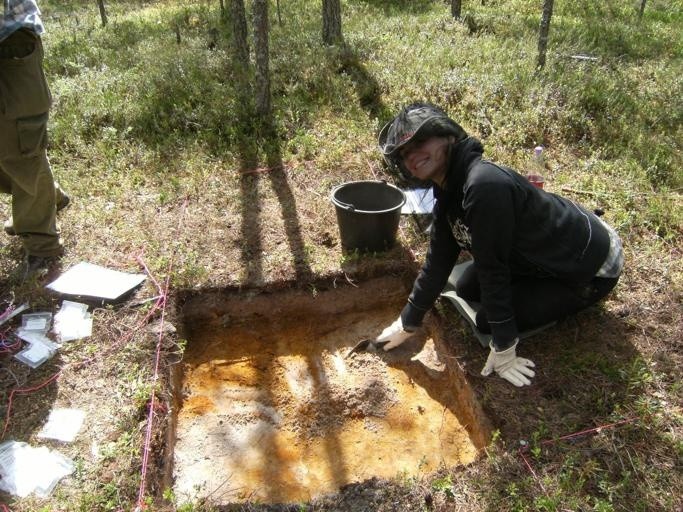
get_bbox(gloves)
[376,315,415,351]
[481,337,536,387]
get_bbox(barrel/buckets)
[331,178,407,249]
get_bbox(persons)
[370,99,625,390]
[0,1,71,265]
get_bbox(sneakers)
[3,181,70,284]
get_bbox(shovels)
[343,340,381,363]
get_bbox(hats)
[379,106,468,156]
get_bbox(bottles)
[528,145,546,189]
